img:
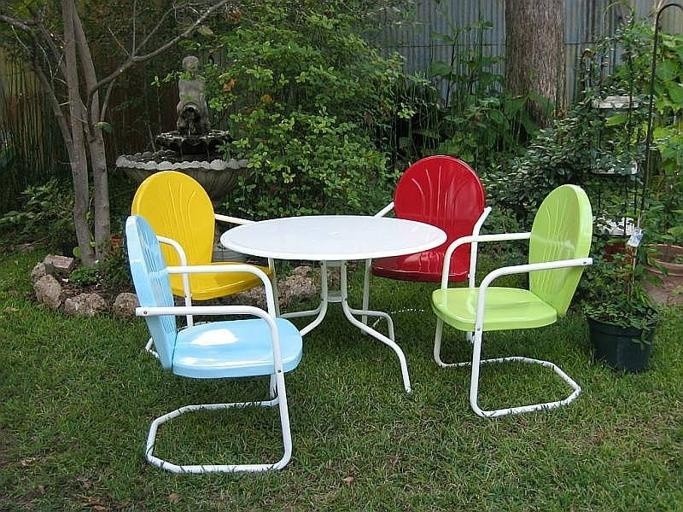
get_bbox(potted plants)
[582,204,675,371]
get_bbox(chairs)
[124,215,305,472]
[434,184,596,418]
[356,155,496,345]
[130,169,275,360]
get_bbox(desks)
[220,215,448,400]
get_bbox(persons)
[176,55,211,131]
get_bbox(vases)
[639,243,683,306]
